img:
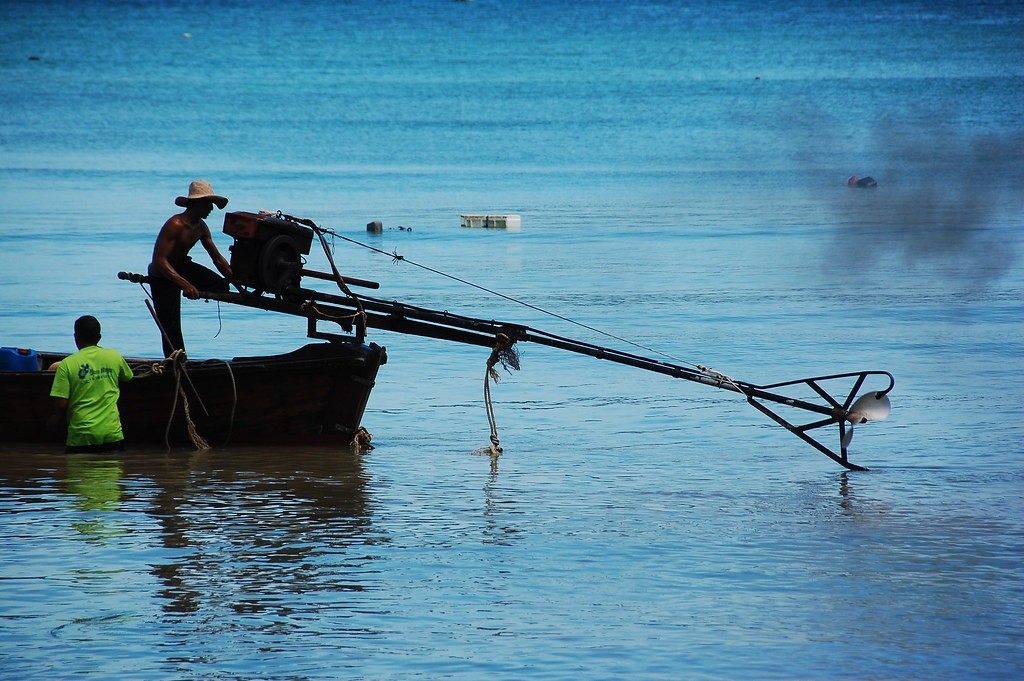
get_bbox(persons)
[49,315,134,455]
[147,180,239,361]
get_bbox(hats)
[175,178,229,210]
